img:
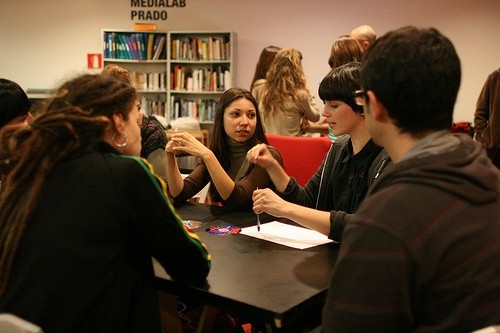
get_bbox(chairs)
[267,134,332,188]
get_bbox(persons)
[475,68,500,171]
[165,88,283,209]
[319,25,500,333]
[250,45,320,137]
[0,63,211,333]
[245,61,390,245]
[328,25,377,144]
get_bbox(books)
[105,32,230,120]
[132,23,157,31]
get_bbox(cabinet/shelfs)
[102,29,237,131]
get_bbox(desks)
[152,201,334,333]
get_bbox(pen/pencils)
[257,187,261,232]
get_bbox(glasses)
[353,89,367,106]
